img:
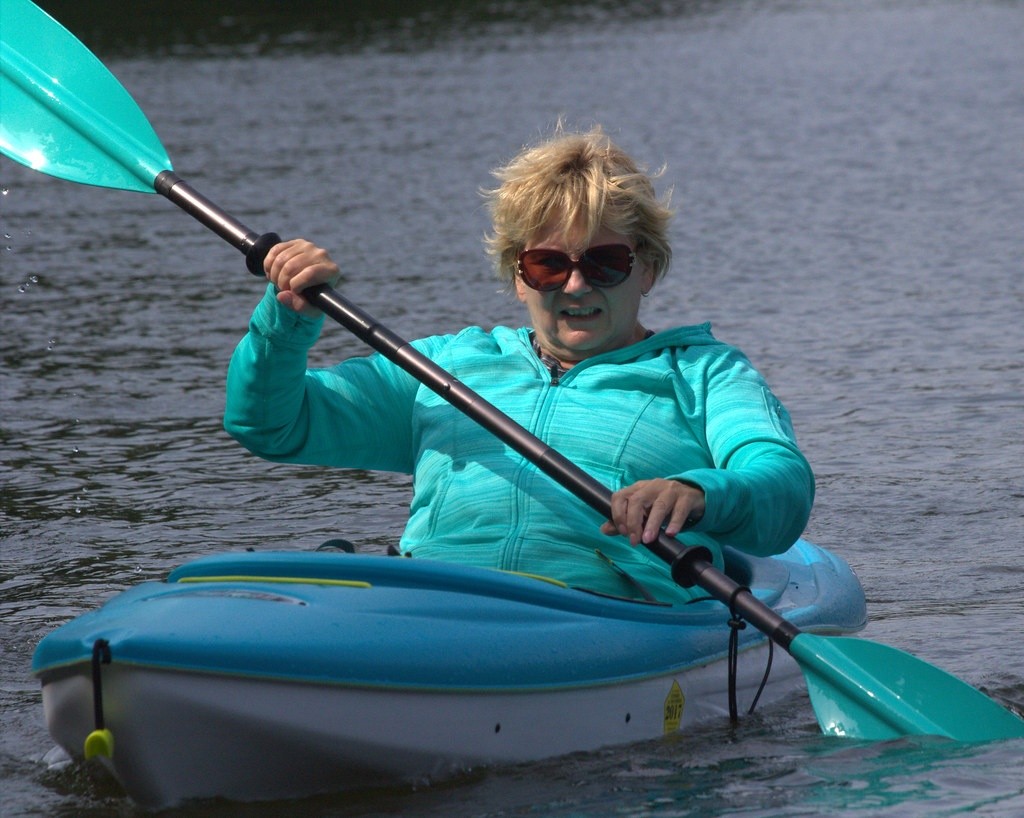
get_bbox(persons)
[222,118,817,611]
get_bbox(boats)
[27,532,868,812]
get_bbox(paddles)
[2,0,1023,745]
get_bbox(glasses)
[516,244,639,291]
